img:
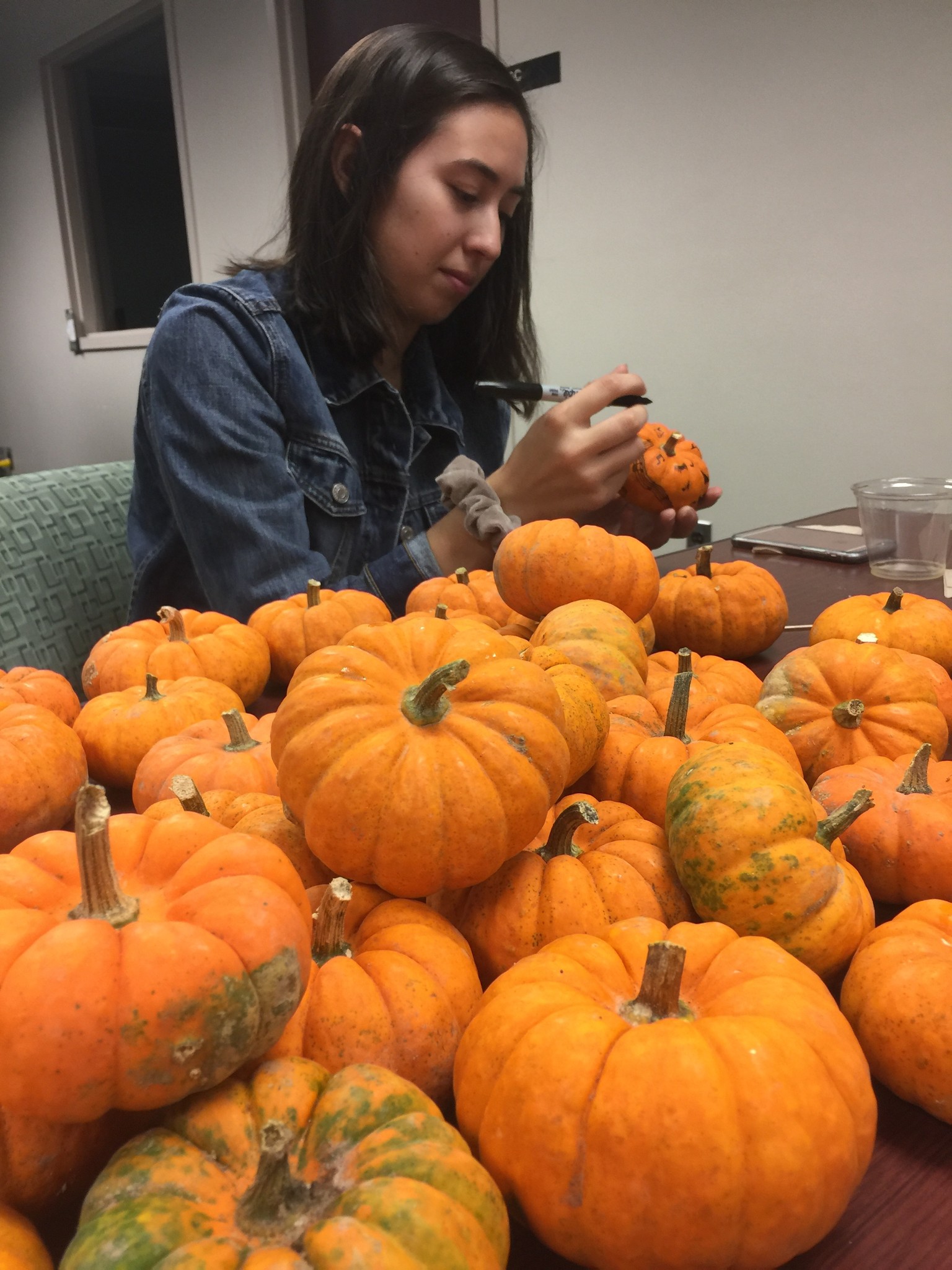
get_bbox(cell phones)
[730,524,898,564]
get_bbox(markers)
[474,381,653,408]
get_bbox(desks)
[71,505,952,1270]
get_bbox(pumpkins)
[617,422,708,516]
[0,516,952,1269]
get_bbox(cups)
[852,477,952,581]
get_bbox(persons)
[124,27,721,697]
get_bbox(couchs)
[0,460,137,708]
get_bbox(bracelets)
[436,453,522,555]
